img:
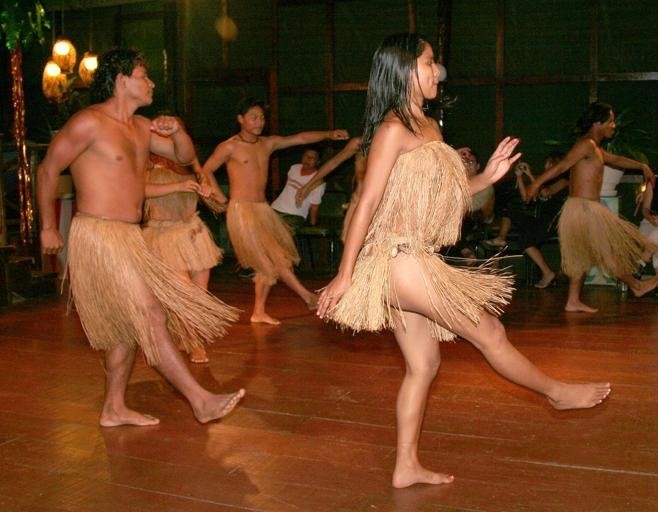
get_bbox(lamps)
[51,0,77,73]
[43,1,68,104]
[78,1,100,84]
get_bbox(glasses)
[462,159,476,167]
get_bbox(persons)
[295,120,470,243]
[484,153,565,290]
[315,33,612,487]
[202,95,350,325]
[236,145,326,277]
[525,102,658,313]
[144,109,224,363]
[453,151,496,267]
[36,48,246,426]
[634,173,658,276]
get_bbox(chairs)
[489,231,536,287]
[618,174,644,226]
[543,235,560,246]
[302,191,348,280]
